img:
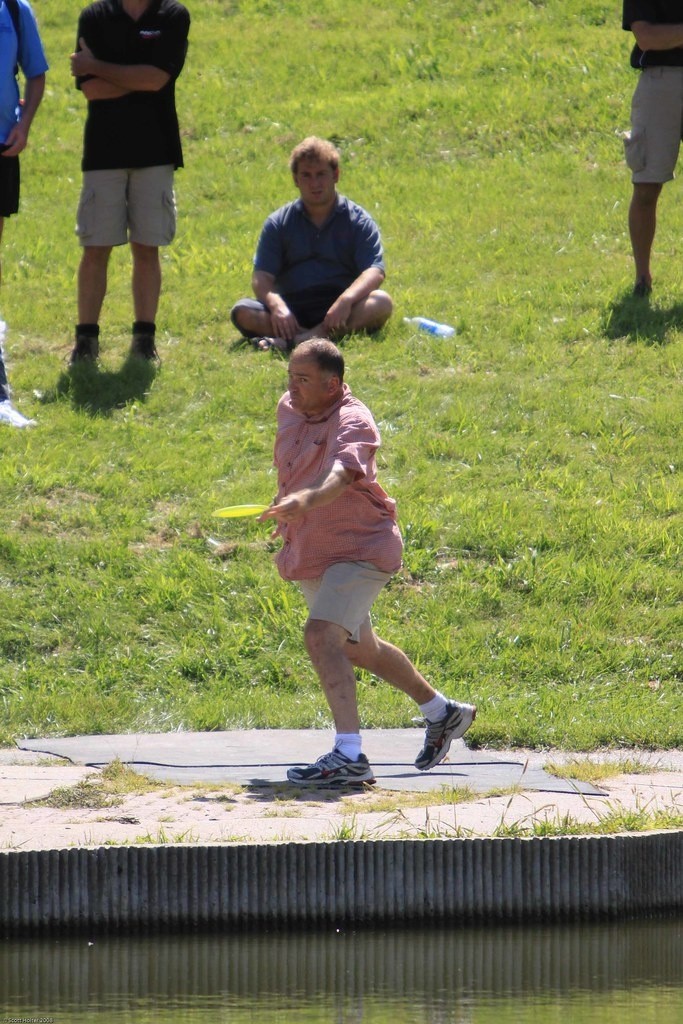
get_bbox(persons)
[69,0,191,360]
[256,338,477,784]
[622,0,683,296]
[0,0,50,430]
[230,135,392,354]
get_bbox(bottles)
[404,315,455,339]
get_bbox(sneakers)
[286,735,376,789]
[416,699,478,769]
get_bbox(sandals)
[253,337,294,356]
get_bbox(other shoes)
[129,333,157,363]
[634,282,652,298]
[68,335,99,365]
[0,401,34,428]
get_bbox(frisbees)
[210,504,270,518]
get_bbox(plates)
[212,506,271,516]
